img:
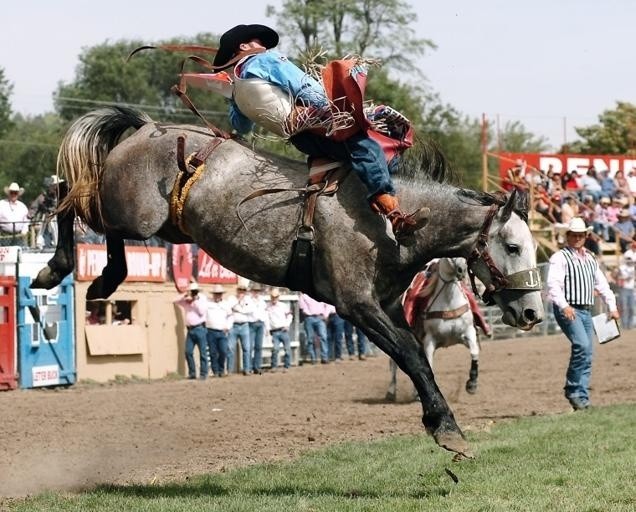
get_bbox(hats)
[187,282,280,298]
[4,182,26,197]
[214,25,279,73]
[49,175,64,184]
[556,218,594,237]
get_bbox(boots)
[372,193,430,236]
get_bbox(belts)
[572,304,594,310]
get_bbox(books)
[589,312,622,344]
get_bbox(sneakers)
[186,354,366,380]
[564,390,590,408]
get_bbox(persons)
[501,160,636,330]
[300,292,377,366]
[545,213,623,414]
[173,283,293,380]
[0,174,104,248]
[211,22,432,244]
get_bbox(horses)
[30,105,545,459]
[385,257,480,402]
